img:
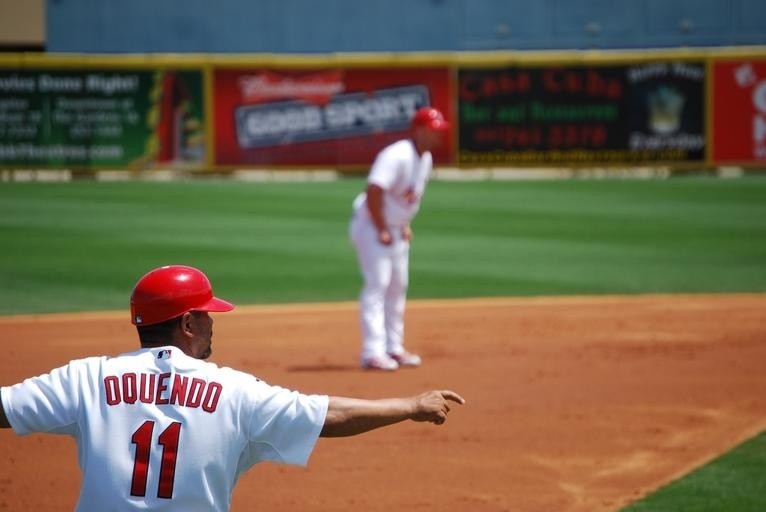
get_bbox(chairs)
[360,354,398,370]
[389,348,421,366]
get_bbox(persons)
[0,265,465,512]
[349,107,452,371]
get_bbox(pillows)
[411,109,449,130]
[130,265,233,325]
